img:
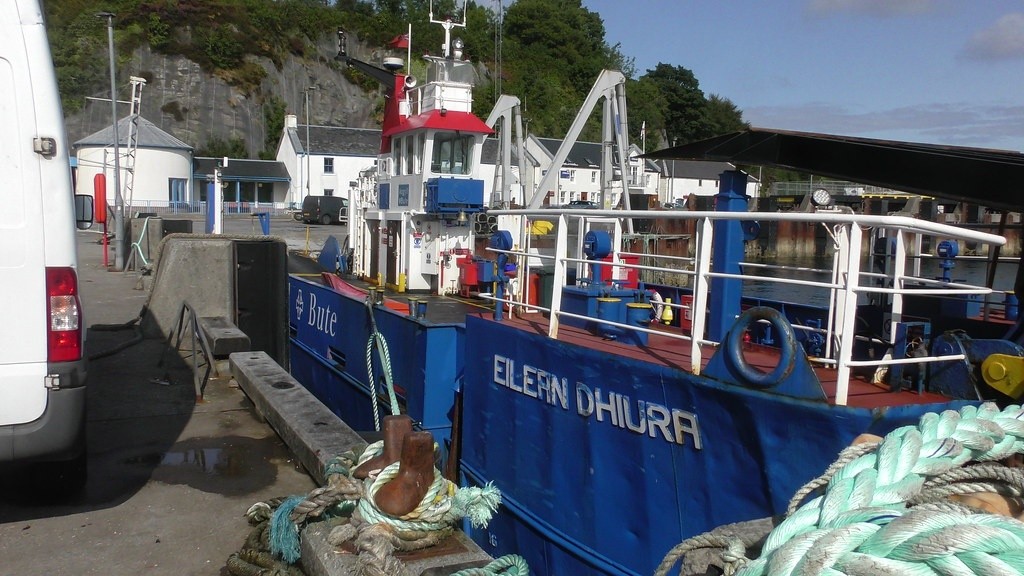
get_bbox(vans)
[302,196,349,226]
[1,0,94,504]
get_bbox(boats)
[287,1,1024,576]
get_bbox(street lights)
[303,87,316,193]
[95,11,125,274]
[671,135,678,203]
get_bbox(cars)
[562,200,599,210]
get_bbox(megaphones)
[403,74,417,88]
